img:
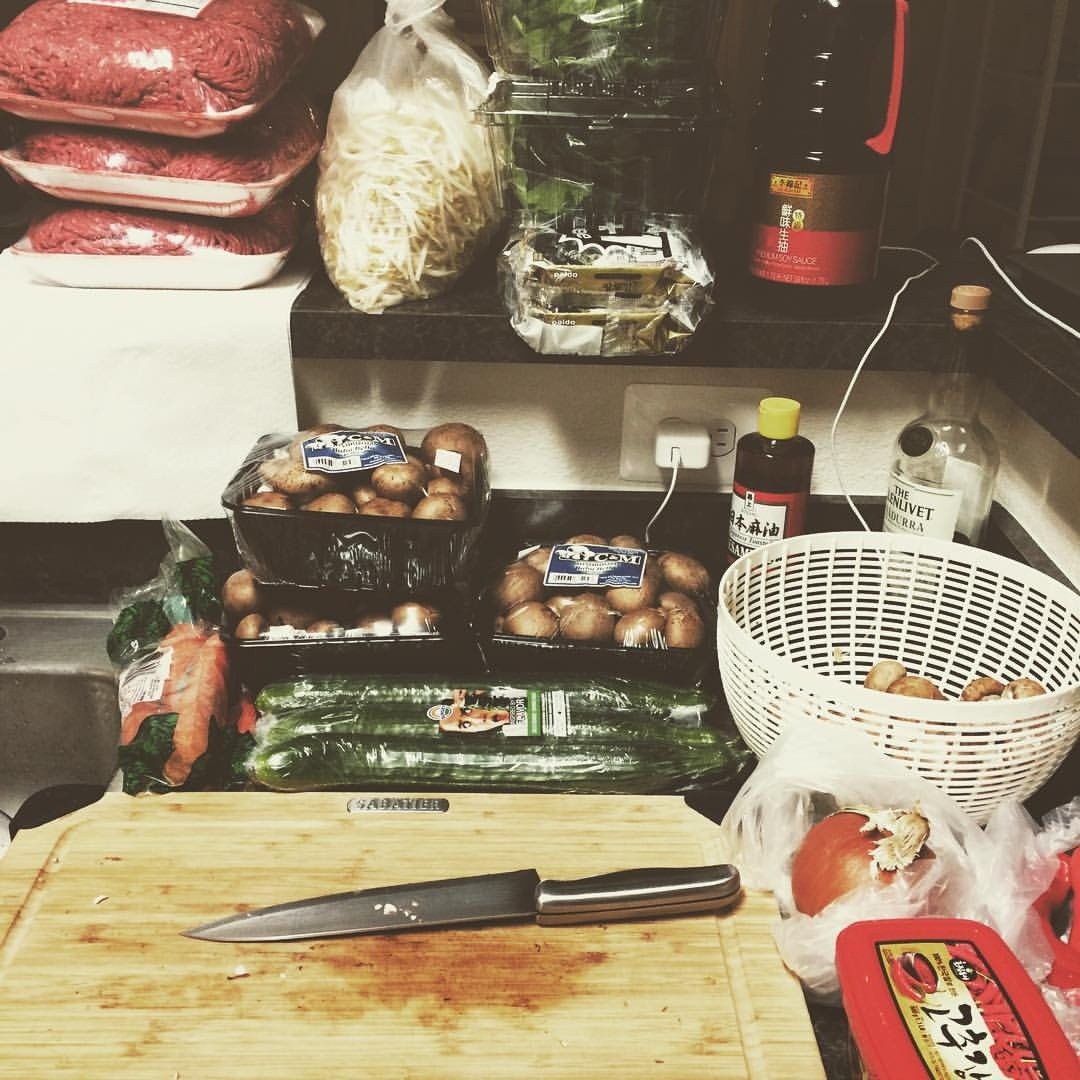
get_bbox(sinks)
[2,604,139,850]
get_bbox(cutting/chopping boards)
[3,786,824,1080]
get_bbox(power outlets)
[614,381,773,488]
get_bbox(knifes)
[183,858,739,945]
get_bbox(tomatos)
[790,812,935,919]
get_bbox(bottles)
[751,0,909,305]
[879,286,1004,552]
[725,394,815,577]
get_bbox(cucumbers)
[250,670,752,794]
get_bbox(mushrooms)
[493,532,710,647]
[241,419,488,523]
[223,566,440,640]
